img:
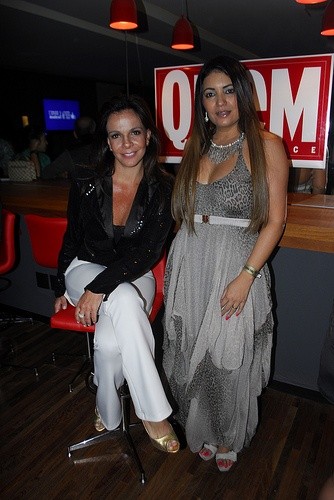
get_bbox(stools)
[0,207,49,357]
[50,247,186,486]
[24,214,93,394]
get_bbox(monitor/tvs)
[38,96,82,133]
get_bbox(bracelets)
[242,264,262,279]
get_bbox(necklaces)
[206,131,245,165]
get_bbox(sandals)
[142,419,180,453]
[94,408,106,432]
[199,444,218,461]
[215,449,237,473]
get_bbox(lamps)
[109,0,334,50]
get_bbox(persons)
[159,56,289,471]
[19,117,101,180]
[54,97,182,452]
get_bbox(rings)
[79,314,85,317]
[232,306,238,311]
